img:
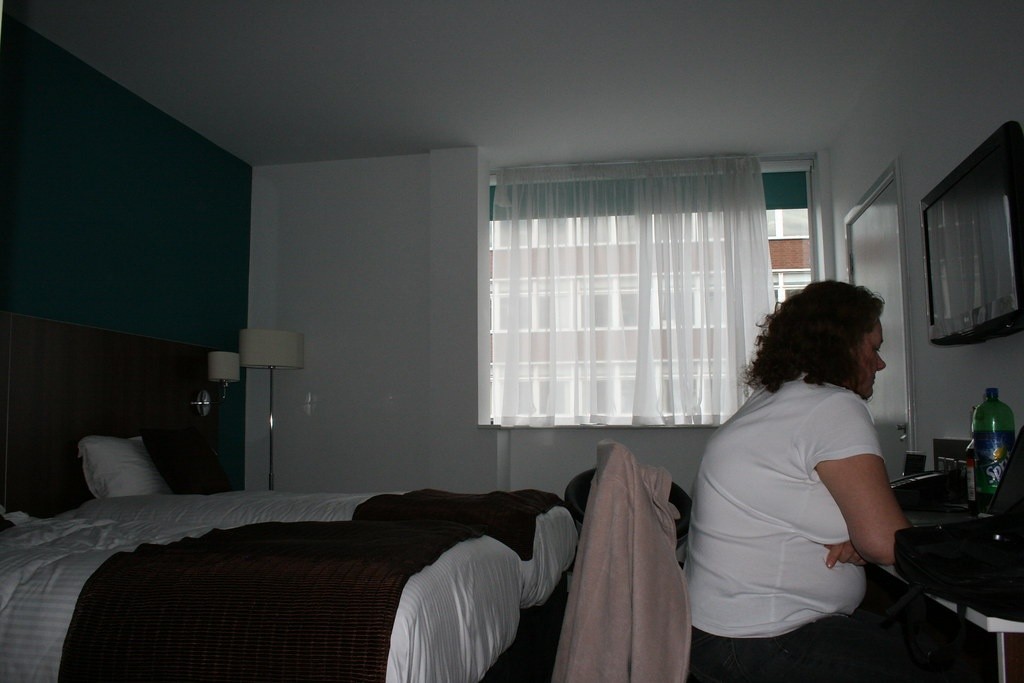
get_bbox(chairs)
[565,465,694,543]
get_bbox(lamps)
[239,328,305,490]
[190,350,241,416]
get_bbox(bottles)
[965,438,977,515]
[972,387,1015,514]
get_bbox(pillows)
[78,434,174,499]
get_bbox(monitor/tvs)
[918,118,1024,346]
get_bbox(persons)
[682,280,998,683]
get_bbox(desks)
[877,509,1024,683]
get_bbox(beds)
[4,314,579,609]
[0,518,524,683]
